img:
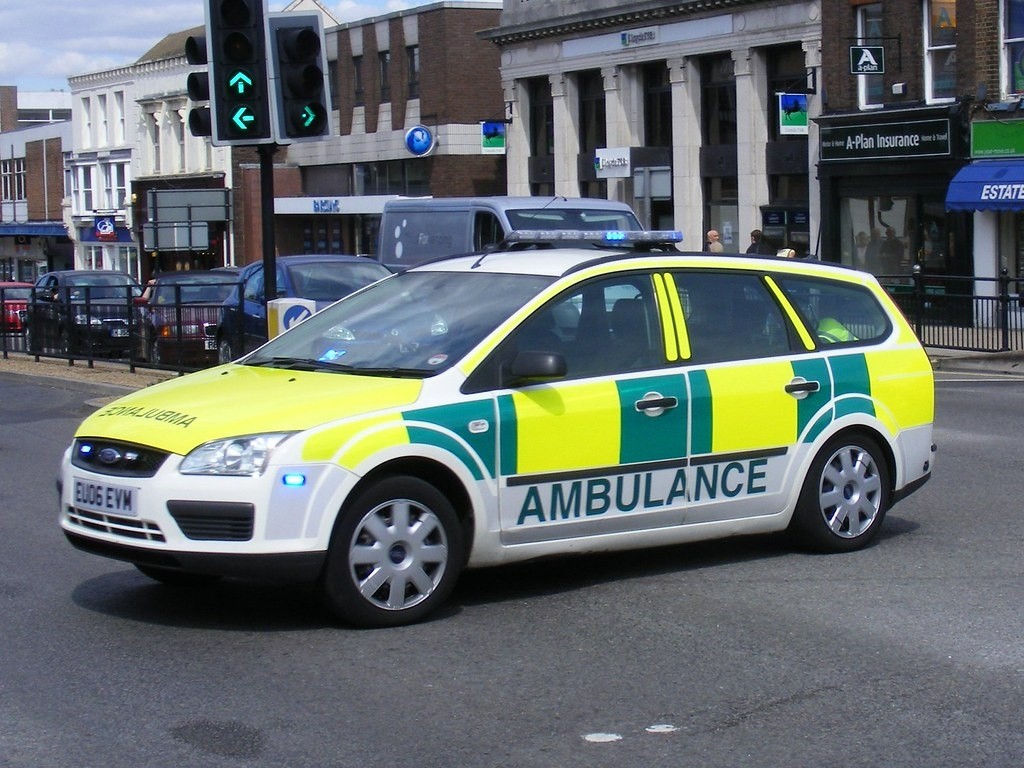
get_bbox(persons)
[705,230,720,253]
[745,229,767,254]
[851,226,906,273]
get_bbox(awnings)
[943,159,1024,215]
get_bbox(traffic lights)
[204,0,275,148]
[185,34,214,137]
[268,12,333,142]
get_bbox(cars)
[25,268,148,355]
[0,280,35,331]
[214,252,399,368]
[55,220,943,629]
[132,270,246,367]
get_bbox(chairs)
[547,297,664,381]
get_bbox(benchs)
[681,286,794,365]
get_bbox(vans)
[376,194,647,278]
